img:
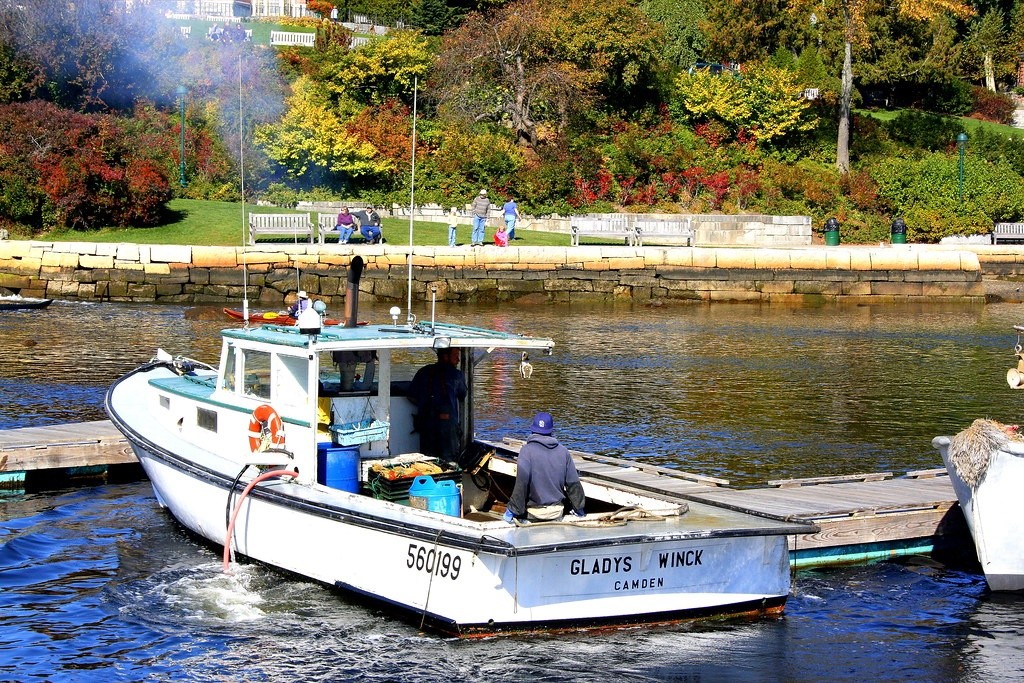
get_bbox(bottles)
[370,418,376,428]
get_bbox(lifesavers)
[249,404,285,468]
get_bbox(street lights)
[956,133,968,201]
[176,86,188,188]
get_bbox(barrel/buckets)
[408,476,460,518]
[317,441,361,493]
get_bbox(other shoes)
[471,243,476,247]
[480,242,484,246]
[339,241,342,244]
[368,239,375,245]
[343,240,347,245]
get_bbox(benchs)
[248,212,317,243]
[318,211,385,244]
[568,213,635,247]
[635,214,698,247]
[990,221,1024,245]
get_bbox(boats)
[223,307,370,327]
[103,319,821,640]
[0,298,54,310]
[933,433,1023,593]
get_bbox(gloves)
[571,509,583,517]
[502,509,514,523]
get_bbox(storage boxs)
[330,416,392,448]
[361,454,468,508]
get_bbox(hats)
[531,413,554,434]
[297,291,309,299]
[367,204,373,209]
[480,189,486,194]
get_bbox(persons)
[337,206,353,245]
[471,190,489,246]
[447,207,459,246]
[350,204,380,245]
[493,225,509,246]
[498,195,522,241]
[504,413,586,523]
[407,347,468,460]
[290,290,309,319]
